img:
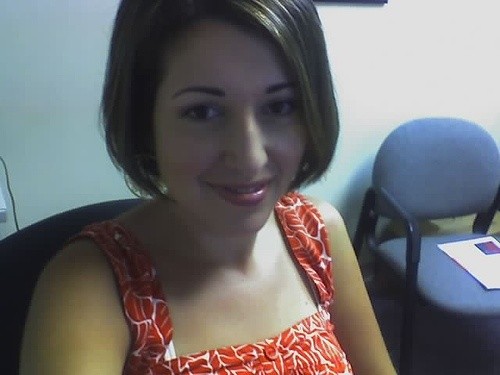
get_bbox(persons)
[18,0,400,374]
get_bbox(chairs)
[351,115,500,374]
